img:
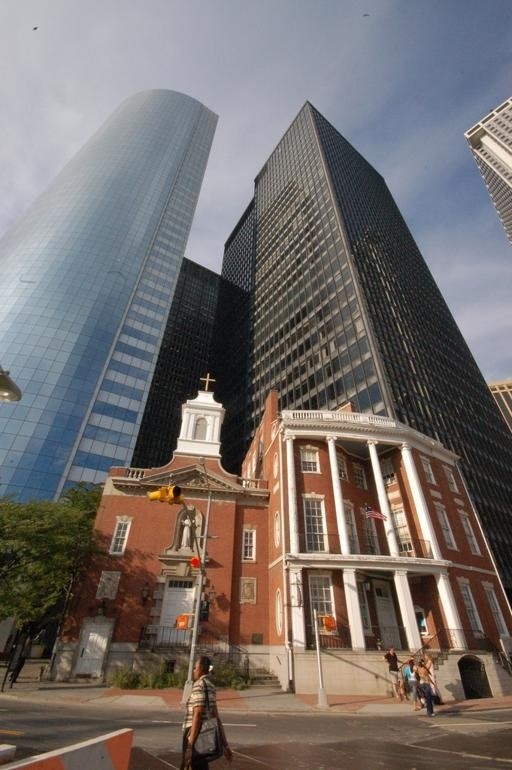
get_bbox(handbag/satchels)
[182,716,224,765]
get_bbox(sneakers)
[427,713,435,717]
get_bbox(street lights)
[178,453,214,709]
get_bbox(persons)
[404,650,445,717]
[179,656,233,769]
[382,647,404,702]
[8,635,33,683]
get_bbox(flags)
[364,500,388,521]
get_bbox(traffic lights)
[147,482,185,507]
